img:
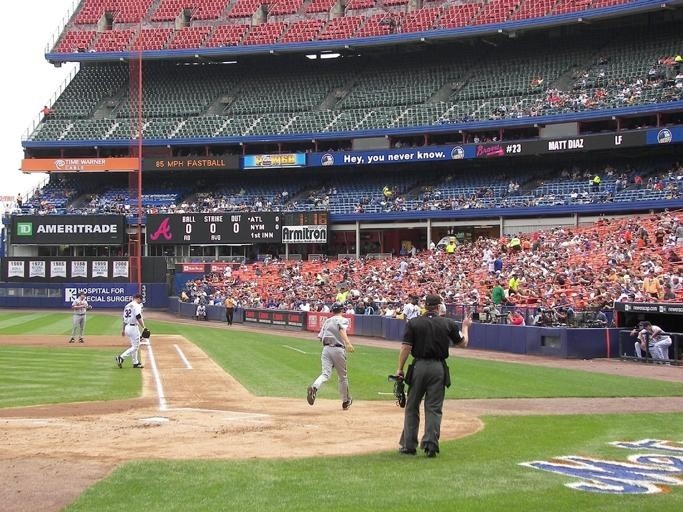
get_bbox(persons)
[18,126,677,218]
[69,292,92,344]
[306,301,355,409]
[399,294,474,457]
[629,321,648,362]
[638,321,672,367]
[184,207,683,328]
[437,52,683,127]
[115,294,147,368]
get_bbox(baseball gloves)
[142,328,150,338]
[87,305,92,311]
[394,382,405,406]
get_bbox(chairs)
[14,1,683,211]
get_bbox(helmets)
[332,302,343,310]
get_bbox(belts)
[325,343,342,347]
[125,323,135,326]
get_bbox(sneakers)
[116,355,123,368]
[427,449,435,457]
[399,448,416,455]
[69,338,75,343]
[307,386,316,405]
[79,339,84,343]
[343,397,352,410]
[133,363,144,368]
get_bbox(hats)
[80,292,87,295]
[426,295,442,306]
